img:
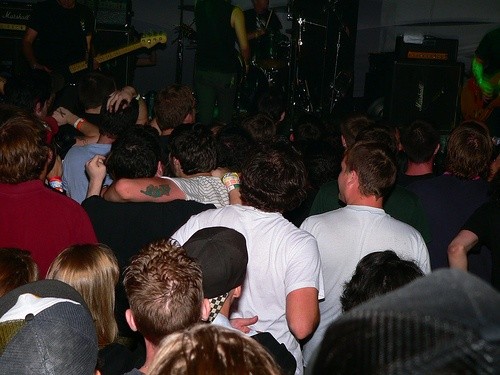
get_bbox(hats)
[182,226,248,324]
[0,279,97,375]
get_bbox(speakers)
[387,60,460,133]
[92,25,137,87]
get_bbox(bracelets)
[74,118,88,131]
[48,176,63,193]
[222,172,240,193]
[135,93,141,101]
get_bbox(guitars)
[68,31,168,76]
[461,73,500,124]
[178,23,245,84]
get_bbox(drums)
[294,80,317,114]
[257,30,281,58]
[254,59,291,94]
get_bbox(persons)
[0,0,500,375]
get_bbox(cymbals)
[270,5,298,12]
[276,40,296,47]
